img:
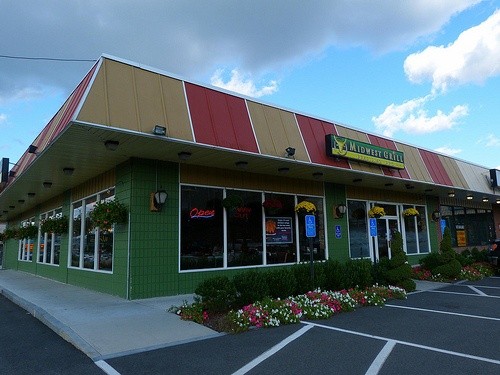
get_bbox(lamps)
[235,161,248,169]
[384,183,500,204]
[154,185,167,210]
[3,145,76,214]
[278,147,363,183]
[154,125,167,135]
[178,152,192,159]
[336,204,346,218]
[432,209,440,222]
[104,140,119,150]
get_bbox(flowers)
[89,197,127,232]
[0,215,68,240]
[368,206,386,217]
[295,201,317,213]
[404,208,419,216]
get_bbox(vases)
[375,214,380,218]
[299,209,311,216]
